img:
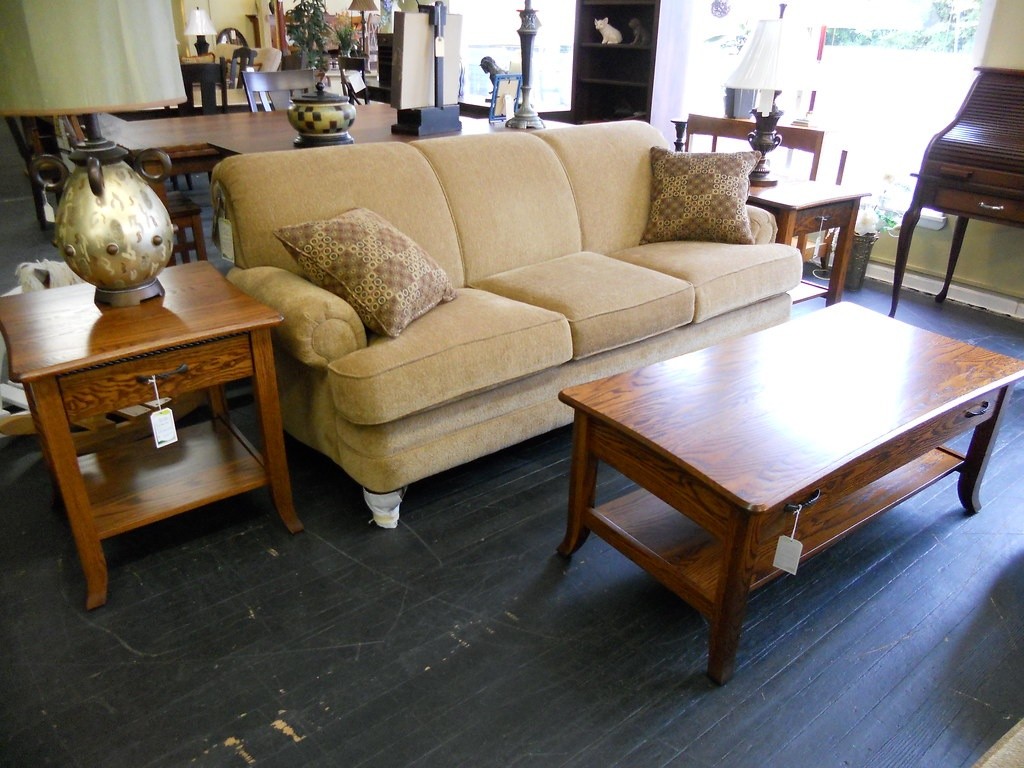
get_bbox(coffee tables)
[554,300,1023,686]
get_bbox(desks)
[84,105,575,267]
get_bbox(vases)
[843,233,880,293]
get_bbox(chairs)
[181,53,215,64]
[337,57,370,105]
[241,69,316,113]
[163,57,227,110]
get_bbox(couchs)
[209,120,804,532]
[212,43,282,88]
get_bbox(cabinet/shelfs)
[377,33,394,86]
[887,66,1024,320]
[685,115,851,184]
[570,0,661,124]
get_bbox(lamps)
[0,0,189,308]
[348,0,378,57]
[183,6,218,55]
[725,18,826,188]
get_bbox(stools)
[166,191,208,267]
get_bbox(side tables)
[749,177,872,309]
[1,259,305,611]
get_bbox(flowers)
[854,208,880,237]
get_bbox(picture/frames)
[488,73,522,121]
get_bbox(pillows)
[639,141,762,246]
[272,206,458,339]
[225,59,262,88]
